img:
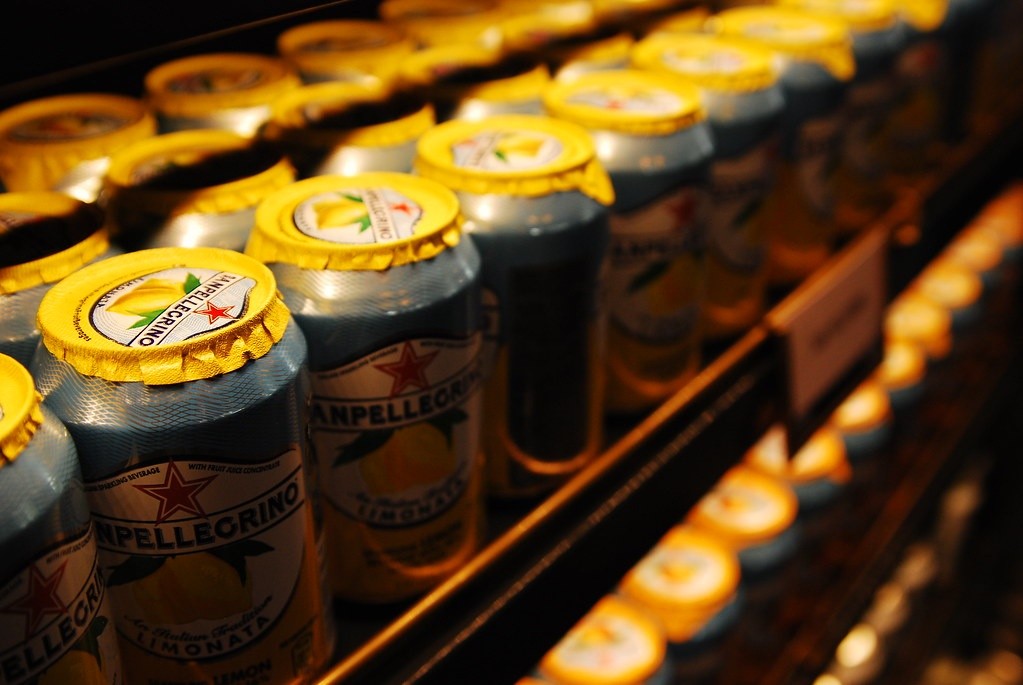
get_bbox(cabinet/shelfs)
[314,94,1023,685]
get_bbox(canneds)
[0,0,1023,685]
